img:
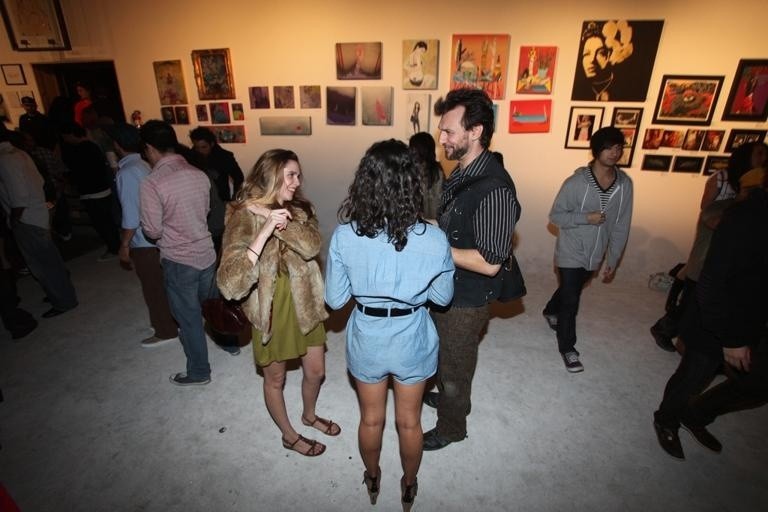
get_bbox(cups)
[105,151,118,168]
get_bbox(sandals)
[281,433,326,457]
[301,412,341,436]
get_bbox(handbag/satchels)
[495,254,528,303]
[201,298,253,347]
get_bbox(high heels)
[364,466,381,505]
[400,474,418,512]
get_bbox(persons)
[571,25,648,101]
[410,102,422,133]
[112,122,179,345]
[0,120,79,319]
[137,116,241,386]
[323,139,455,512]
[405,42,432,86]
[577,115,594,140]
[422,88,520,451]
[650,138,767,459]
[542,128,633,372]
[179,127,240,218]
[9,93,150,257]
[222,146,341,457]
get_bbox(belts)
[356,302,421,318]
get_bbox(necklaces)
[587,76,615,100]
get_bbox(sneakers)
[139,334,180,348]
[561,351,584,373]
[220,347,240,356]
[169,372,212,386]
[543,308,558,332]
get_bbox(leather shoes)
[43,297,49,302]
[680,422,723,454]
[422,391,471,416]
[650,326,677,352]
[653,421,685,461]
[42,301,79,318]
[422,426,466,451]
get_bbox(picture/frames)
[610,107,644,168]
[191,48,237,101]
[0,0,73,52]
[564,107,605,149]
[723,128,768,152]
[720,58,768,122]
[651,73,725,125]
[1,64,27,85]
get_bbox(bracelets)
[247,246,260,259]
